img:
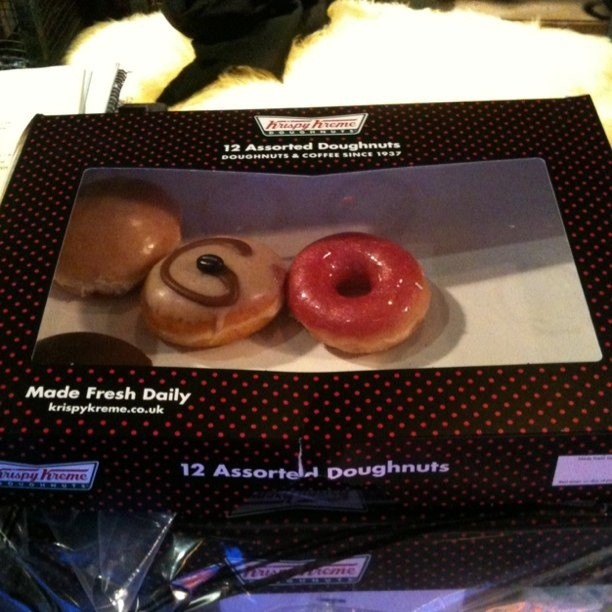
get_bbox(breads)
[136,234,286,348]
[32,335,151,365]
[54,174,181,298]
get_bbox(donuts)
[286,232,433,355]
